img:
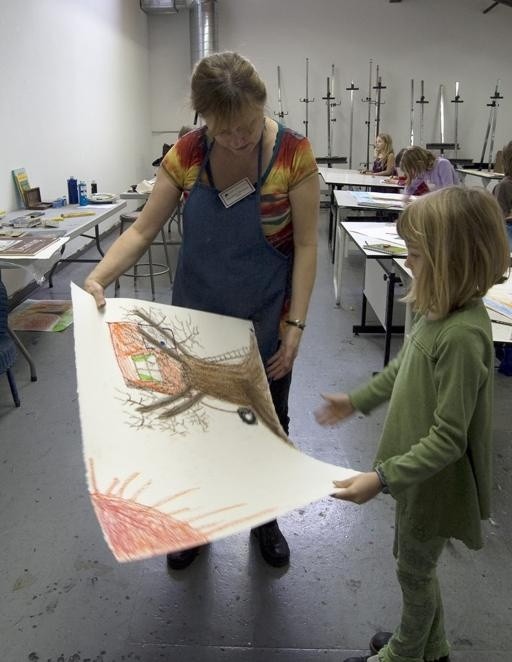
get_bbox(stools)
[116,212,174,302]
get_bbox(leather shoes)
[343,631,450,662]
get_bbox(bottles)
[67,176,97,206]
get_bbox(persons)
[83,52,318,571]
[492,139,512,251]
[360,132,400,221]
[400,145,462,203]
[382,148,429,195]
[310,185,510,662]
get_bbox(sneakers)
[251,520,290,568]
[166,547,200,570]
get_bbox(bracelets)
[374,468,391,494]
[285,320,305,330]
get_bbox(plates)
[87,192,118,203]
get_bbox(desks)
[0,197,129,314]
[319,164,447,382]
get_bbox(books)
[361,243,409,256]
[13,169,31,206]
[0,211,70,260]
